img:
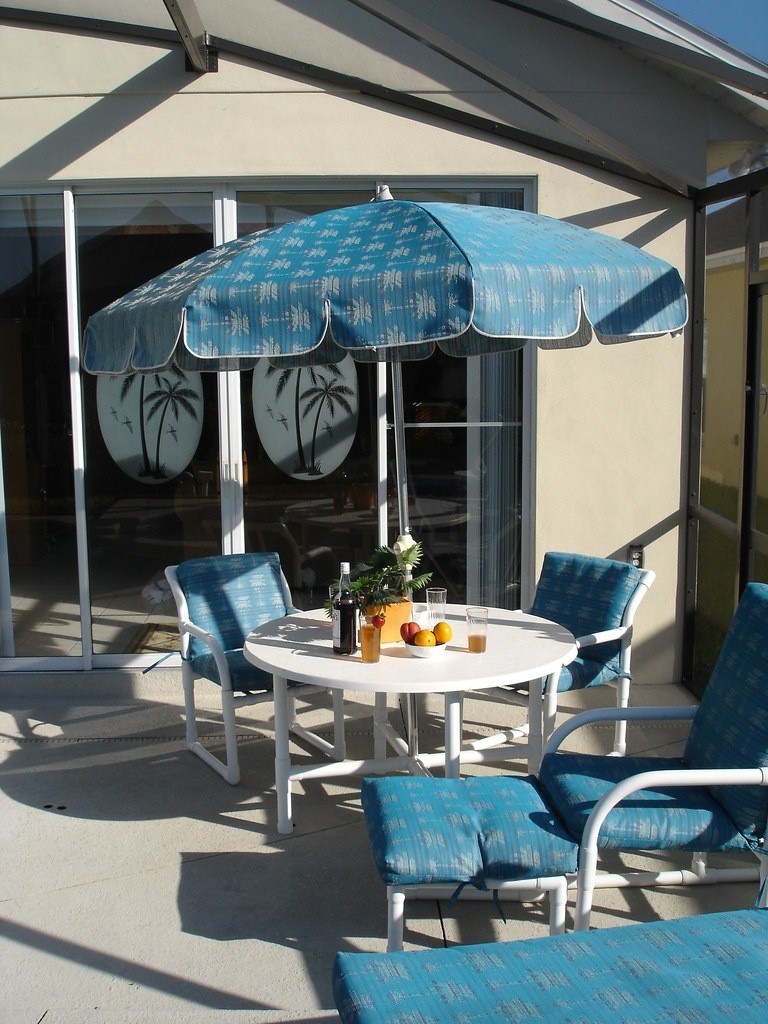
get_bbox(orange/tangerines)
[415,621,452,646]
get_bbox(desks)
[243,598,580,834]
[283,490,470,602]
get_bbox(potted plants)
[322,540,435,644]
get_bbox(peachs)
[400,622,421,643]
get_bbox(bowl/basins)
[405,642,447,657]
[370,506,396,517]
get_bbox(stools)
[361,774,583,957]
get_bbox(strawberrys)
[372,613,386,628]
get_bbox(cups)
[465,607,489,653]
[347,483,354,503]
[332,490,345,515]
[408,486,416,505]
[359,615,381,663]
[426,587,447,623]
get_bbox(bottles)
[331,561,358,654]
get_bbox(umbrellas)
[81,185,691,759]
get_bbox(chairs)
[329,871,768,1024]
[163,552,346,787]
[274,521,336,606]
[540,582,768,936]
[452,550,655,762]
